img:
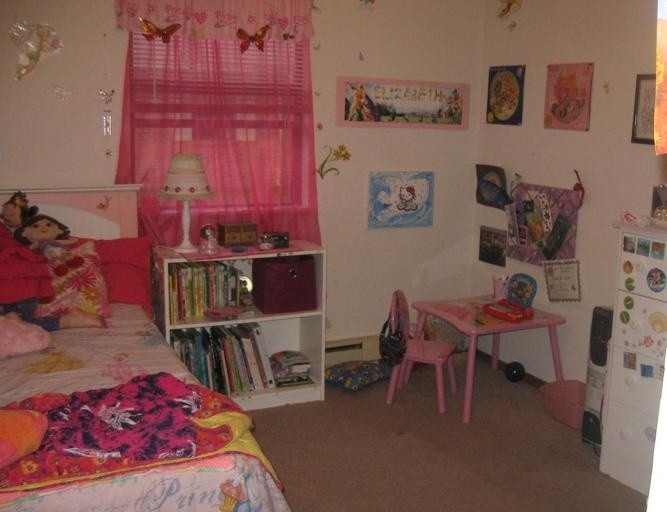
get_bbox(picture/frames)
[625,70,658,146]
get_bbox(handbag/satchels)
[379,293,408,365]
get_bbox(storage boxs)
[538,379,588,431]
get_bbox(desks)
[401,290,571,424]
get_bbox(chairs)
[381,288,459,416]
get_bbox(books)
[169,260,320,396]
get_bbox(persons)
[0,190,112,318]
[0,220,109,331]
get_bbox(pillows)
[0,408,50,471]
[0,310,53,361]
[320,357,391,395]
[39,234,156,321]
[31,237,115,322]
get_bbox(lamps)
[156,154,216,254]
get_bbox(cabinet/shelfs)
[597,220,667,499]
[143,231,329,416]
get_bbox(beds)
[1,177,297,512]
